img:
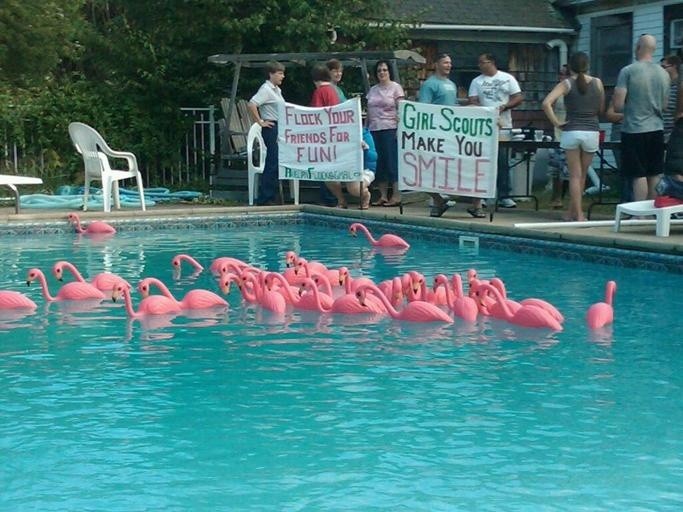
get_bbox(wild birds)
[55,260,131,295]
[65,212,116,235]
[110,282,181,315]
[1,290,34,310]
[585,280,619,330]
[205,248,566,334]
[28,268,108,301]
[140,277,231,310]
[349,221,408,250]
[172,252,203,269]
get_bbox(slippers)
[431,205,448,217]
[383,199,401,206]
[467,208,485,217]
[372,198,386,206]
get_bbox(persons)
[467,51,523,208]
[303,58,378,210]
[248,60,294,205]
[544,64,569,209]
[418,53,456,207]
[542,50,606,221]
[605,33,683,223]
[424,192,486,219]
[362,60,405,208]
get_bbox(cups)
[535,130,544,142]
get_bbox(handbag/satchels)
[656,177,671,195]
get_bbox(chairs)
[66,120,147,214]
[613,196,683,237]
[246,118,300,205]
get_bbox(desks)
[494,137,628,221]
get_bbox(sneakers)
[429,195,456,207]
[496,198,516,208]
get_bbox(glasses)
[661,65,672,69]
